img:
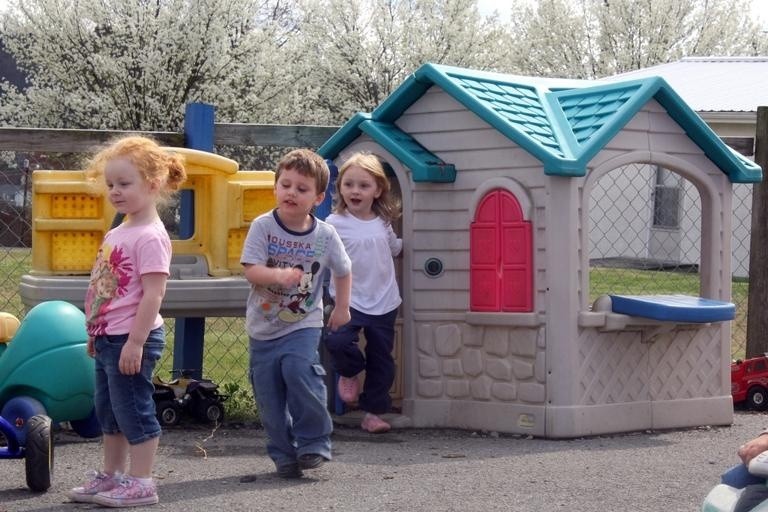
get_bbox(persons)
[239,150,353,477]
[66,135,187,506]
[319,150,403,432]
[736,429,768,466]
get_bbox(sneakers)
[93,475,159,507]
[69,472,125,502]
[361,416,391,433]
[338,375,360,403]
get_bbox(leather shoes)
[299,454,324,469]
[278,463,304,478]
